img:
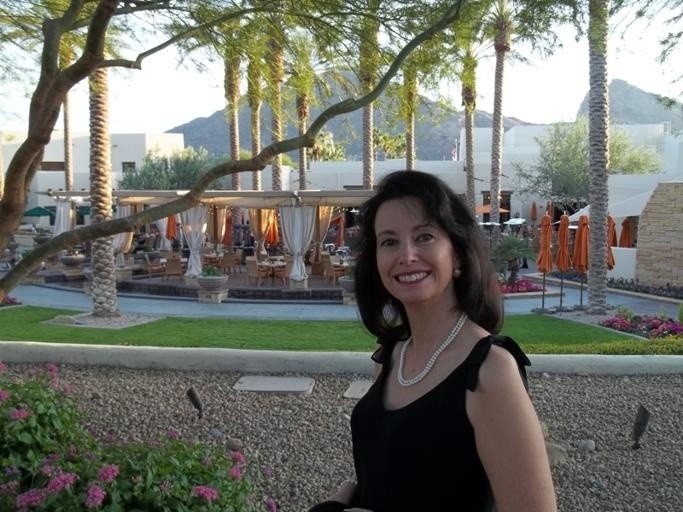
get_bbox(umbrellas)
[555,211,573,307]
[166,215,177,241]
[476,203,510,214]
[607,212,616,247]
[537,211,553,308]
[221,210,234,246]
[531,200,537,222]
[337,212,345,248]
[267,213,281,277]
[571,210,591,305]
[618,217,632,248]
[607,238,615,269]
[547,199,553,222]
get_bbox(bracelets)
[339,476,360,488]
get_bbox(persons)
[306,168,559,512]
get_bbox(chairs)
[123,247,348,289]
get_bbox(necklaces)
[397,311,469,386]
